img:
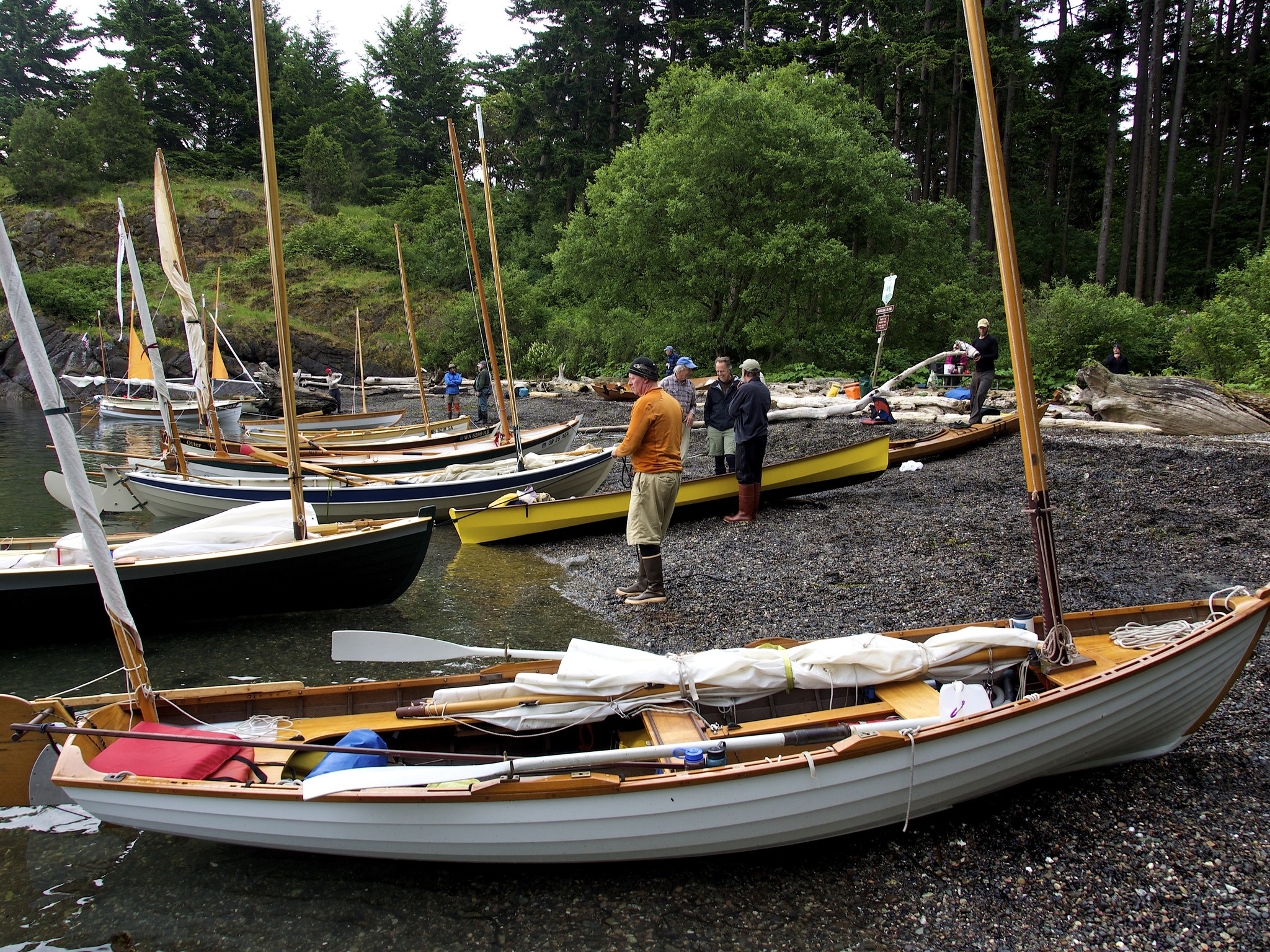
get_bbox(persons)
[474,364,492,422]
[326,368,343,413]
[444,364,462,420]
[663,346,679,376]
[967,318,998,424]
[658,357,698,469]
[944,343,967,389]
[704,356,742,476]
[611,357,683,605]
[476,360,490,424]
[1104,344,1129,375]
[723,358,771,522]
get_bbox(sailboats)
[0,0,1270,867]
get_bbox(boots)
[723,482,762,523]
[477,410,488,424]
[616,544,648,598]
[455,411,460,419]
[473,409,482,422]
[447,411,452,420]
[624,544,668,606]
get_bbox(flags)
[81,332,89,349]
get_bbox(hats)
[676,356,698,369]
[977,318,989,329]
[1112,343,1120,350]
[326,368,331,374]
[628,356,659,382]
[739,358,761,373]
[663,345,673,351]
[446,363,457,371]
[477,364,480,367]
[478,360,487,365]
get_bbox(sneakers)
[949,420,972,429]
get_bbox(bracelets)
[689,412,694,414]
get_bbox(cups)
[954,369,957,374]
[965,370,967,374]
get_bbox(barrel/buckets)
[944,364,951,375]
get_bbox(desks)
[933,374,1007,391]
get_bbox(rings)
[691,422,692,424]
